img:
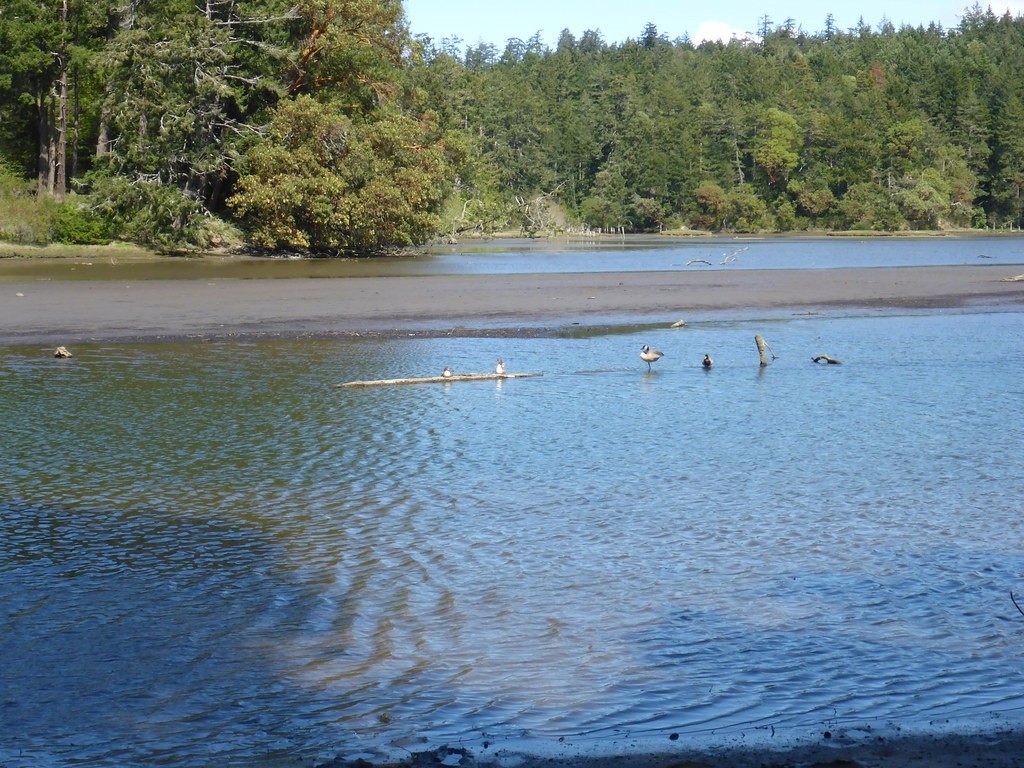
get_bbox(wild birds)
[441,367,454,377]
[496,359,506,374]
[639,345,665,374]
[701,354,713,368]
[813,355,843,365]
